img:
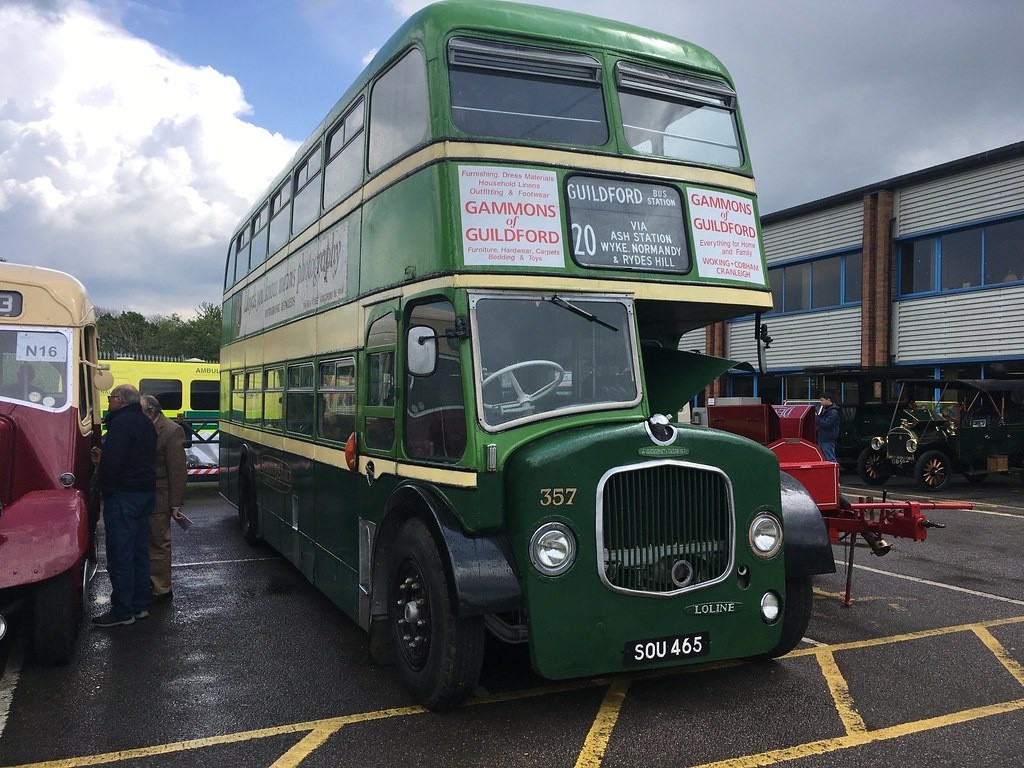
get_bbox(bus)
[218,1,836,714]
[0,259,114,673]
[58,359,282,483]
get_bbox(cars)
[857,377,1023,492]
[803,365,916,475]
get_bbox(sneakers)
[135,611,149,618]
[91,611,135,627]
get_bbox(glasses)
[108,395,123,402]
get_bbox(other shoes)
[149,591,174,606]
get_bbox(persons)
[87,385,187,626]
[814,393,842,463]
[7,363,46,396]
